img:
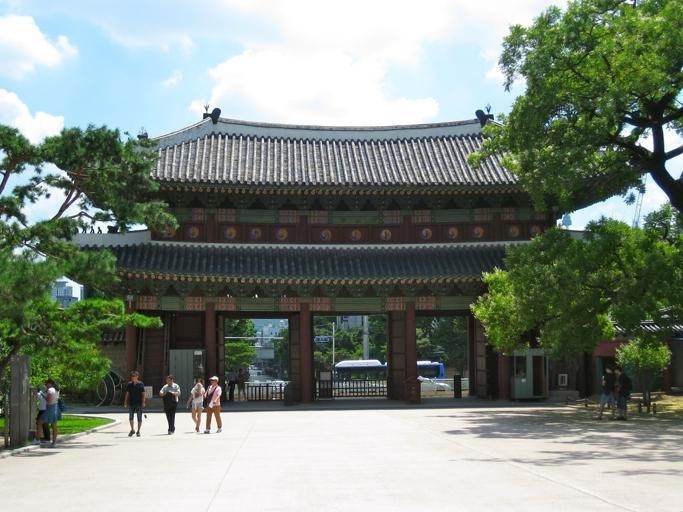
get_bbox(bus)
[332,360,444,381]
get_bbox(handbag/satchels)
[203,396,210,408]
[163,392,177,412]
[58,397,65,411]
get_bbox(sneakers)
[32,437,56,448]
[129,430,140,436]
[595,415,627,421]
[168,427,175,434]
[196,427,222,433]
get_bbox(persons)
[597,365,616,420]
[123,370,146,437]
[159,375,181,435]
[612,367,632,421]
[33,389,50,441]
[225,368,238,402]
[204,375,223,433]
[237,368,247,401]
[31,379,60,448]
[186,378,206,432]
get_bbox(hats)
[209,376,219,382]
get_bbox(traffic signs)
[313,337,329,343]
